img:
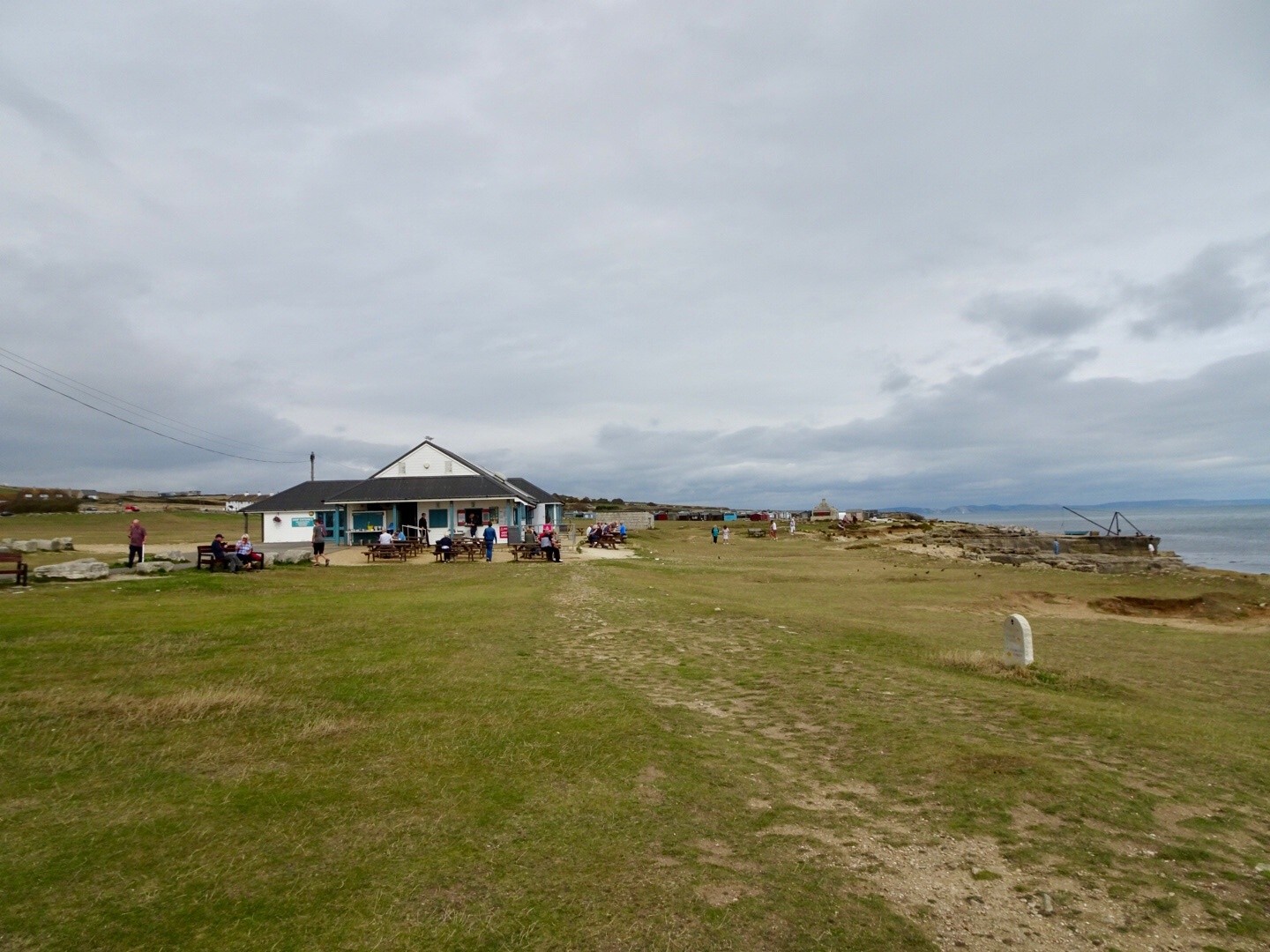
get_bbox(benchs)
[197,545,264,572]
[566,531,584,536]
[360,543,547,563]
[582,537,627,550]
[0,549,28,586]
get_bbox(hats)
[216,534,224,538]
[313,519,318,521]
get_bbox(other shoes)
[325,558,330,566]
[313,563,320,566]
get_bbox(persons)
[437,513,498,563]
[769,516,795,541]
[312,519,330,567]
[711,525,730,545]
[1053,539,1060,554]
[211,534,248,573]
[518,516,563,563]
[1148,541,1155,559]
[586,522,627,548]
[124,519,146,569]
[375,513,432,558]
[235,535,253,570]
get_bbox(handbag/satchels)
[320,524,326,536]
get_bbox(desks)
[566,528,584,536]
[364,537,561,562]
[594,531,623,548]
[345,531,382,546]
